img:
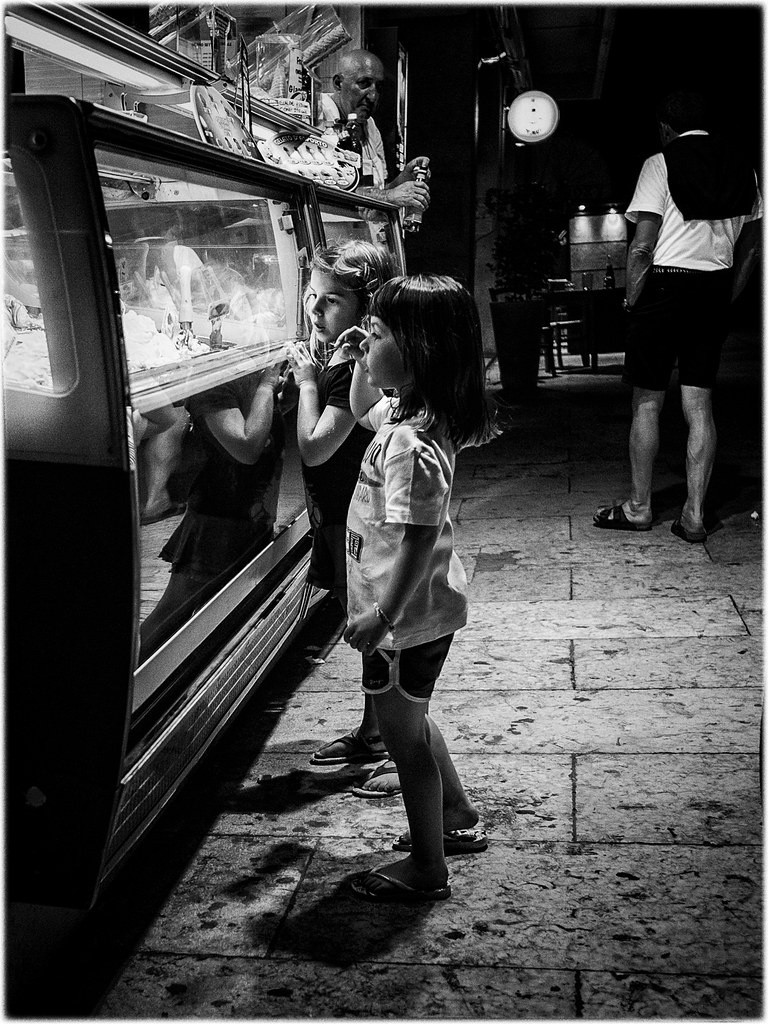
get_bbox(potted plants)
[479,181,551,399]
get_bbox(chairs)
[549,273,598,377]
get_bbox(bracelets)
[375,600,391,628]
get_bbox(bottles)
[402,170,426,232]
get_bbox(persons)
[282,240,403,798]
[328,271,499,902]
[120,258,296,613]
[591,114,765,542]
[310,47,431,213]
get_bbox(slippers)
[392,824,487,854]
[670,517,707,543]
[351,861,452,903]
[141,503,189,525]
[593,503,653,531]
[310,732,391,764]
[354,758,402,796]
